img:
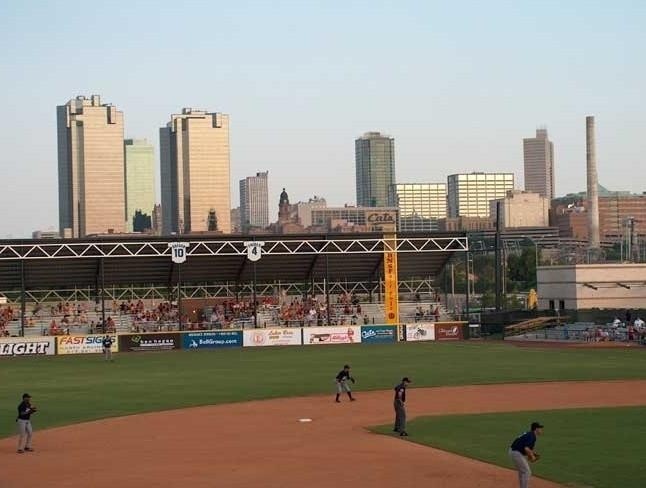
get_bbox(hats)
[531,422,542,430]
[402,377,409,381]
[343,364,351,367]
[22,393,31,397]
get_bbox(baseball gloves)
[528,453,542,464]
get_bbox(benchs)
[518,320,635,341]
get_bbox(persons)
[508,422,545,488]
[393,376,413,437]
[415,293,440,315]
[2,298,145,337]
[102,334,114,362]
[334,364,356,402]
[256,292,371,327]
[15,393,41,453]
[583,310,646,344]
[145,297,254,329]
